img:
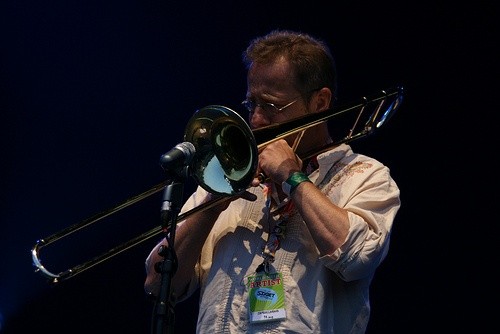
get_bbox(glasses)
[240,96,301,115]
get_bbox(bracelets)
[283,171,311,196]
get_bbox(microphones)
[160,142,196,170]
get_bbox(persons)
[146,30,400,334]
